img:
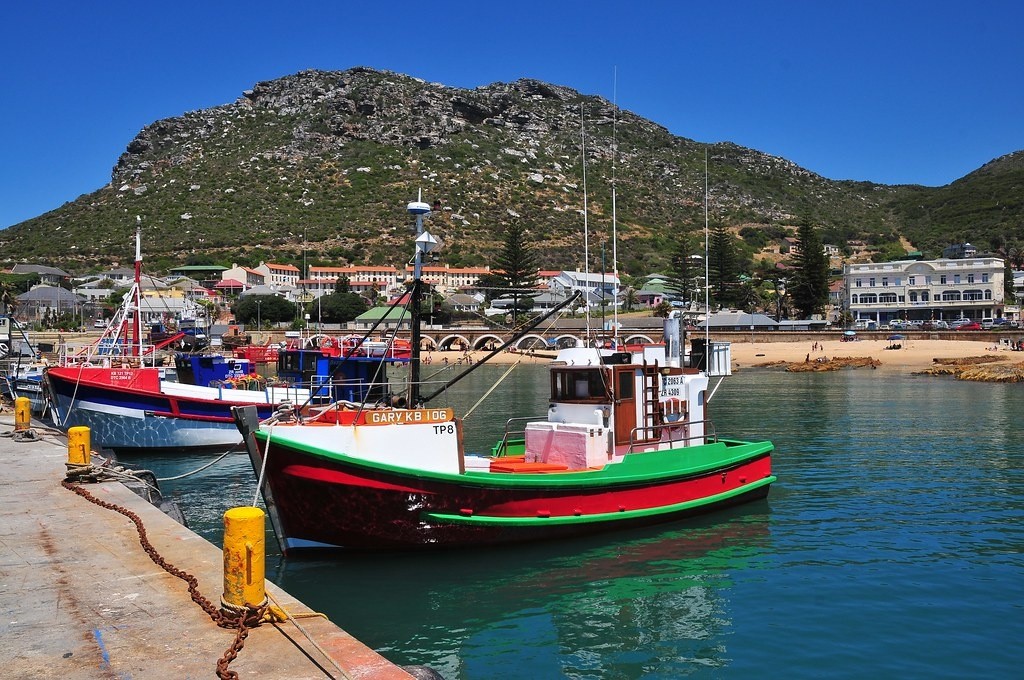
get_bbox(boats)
[0,291,432,425]
[41,215,426,462]
[228,64,777,565]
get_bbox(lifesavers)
[342,334,363,347]
[661,398,686,432]
[322,336,338,349]
[77,361,89,368]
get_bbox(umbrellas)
[886,334,904,341]
[845,331,855,336]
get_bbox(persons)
[805,342,828,366]
[886,344,902,350]
[839,334,858,342]
[985,342,1024,351]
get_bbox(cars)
[853,317,1024,331]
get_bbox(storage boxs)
[464,454,569,474]
